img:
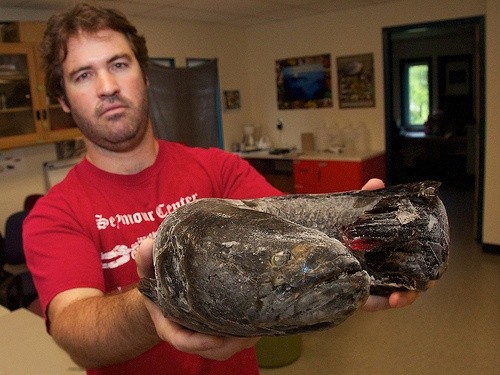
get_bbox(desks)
[234,150,386,194]
[400,132,467,167]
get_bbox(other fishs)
[136,180,450,339]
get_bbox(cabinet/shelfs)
[0,21,85,150]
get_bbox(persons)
[22,3,440,375]
[5,194,42,311]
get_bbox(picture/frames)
[336,53,376,109]
[224,89,241,110]
[275,54,333,109]
